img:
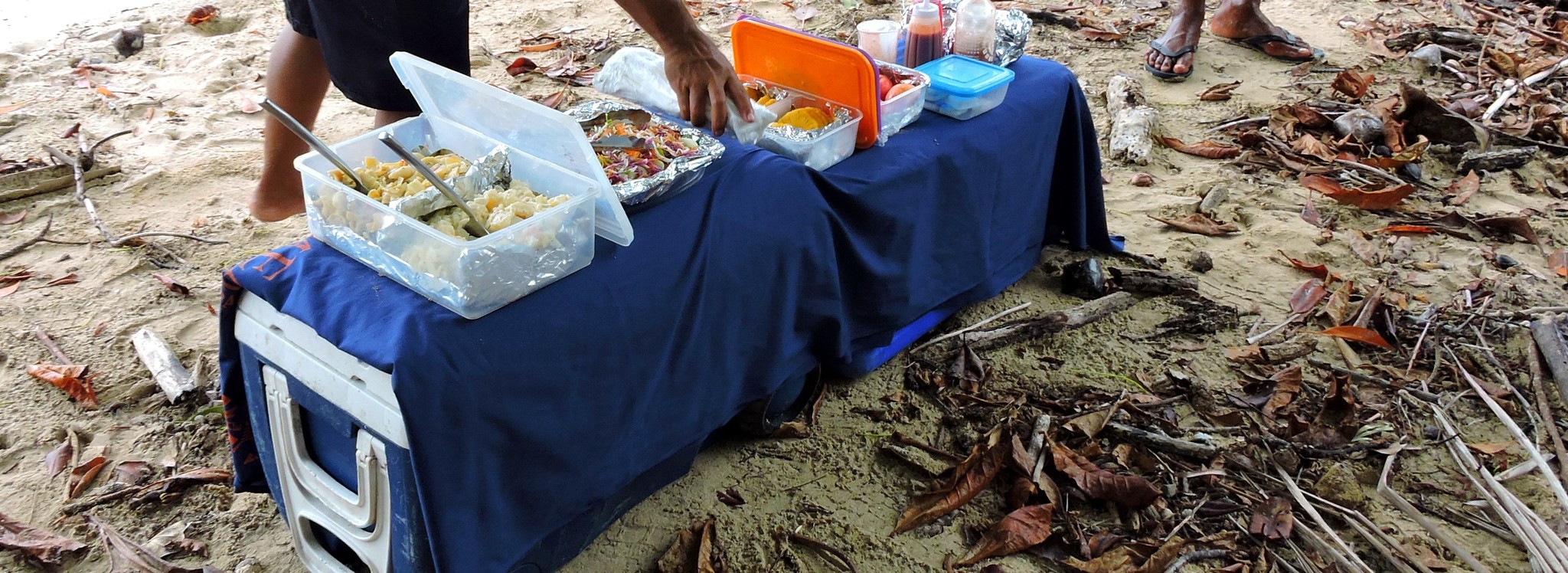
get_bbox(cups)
[856,20,901,63]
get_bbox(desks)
[218,87,836,573]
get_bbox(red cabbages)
[600,128,689,185]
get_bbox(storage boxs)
[291,15,1016,321]
[230,292,433,573]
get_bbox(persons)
[247,0,755,222]
[1146,0,1326,79]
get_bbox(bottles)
[904,0,943,68]
[953,0,996,66]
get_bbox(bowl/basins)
[872,59,931,136]
[913,54,1015,120]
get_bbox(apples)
[878,74,918,102]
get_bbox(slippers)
[1208,26,1324,62]
[1145,40,1198,81]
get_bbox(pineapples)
[774,105,831,132]
[744,87,776,107]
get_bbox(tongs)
[574,108,651,150]
